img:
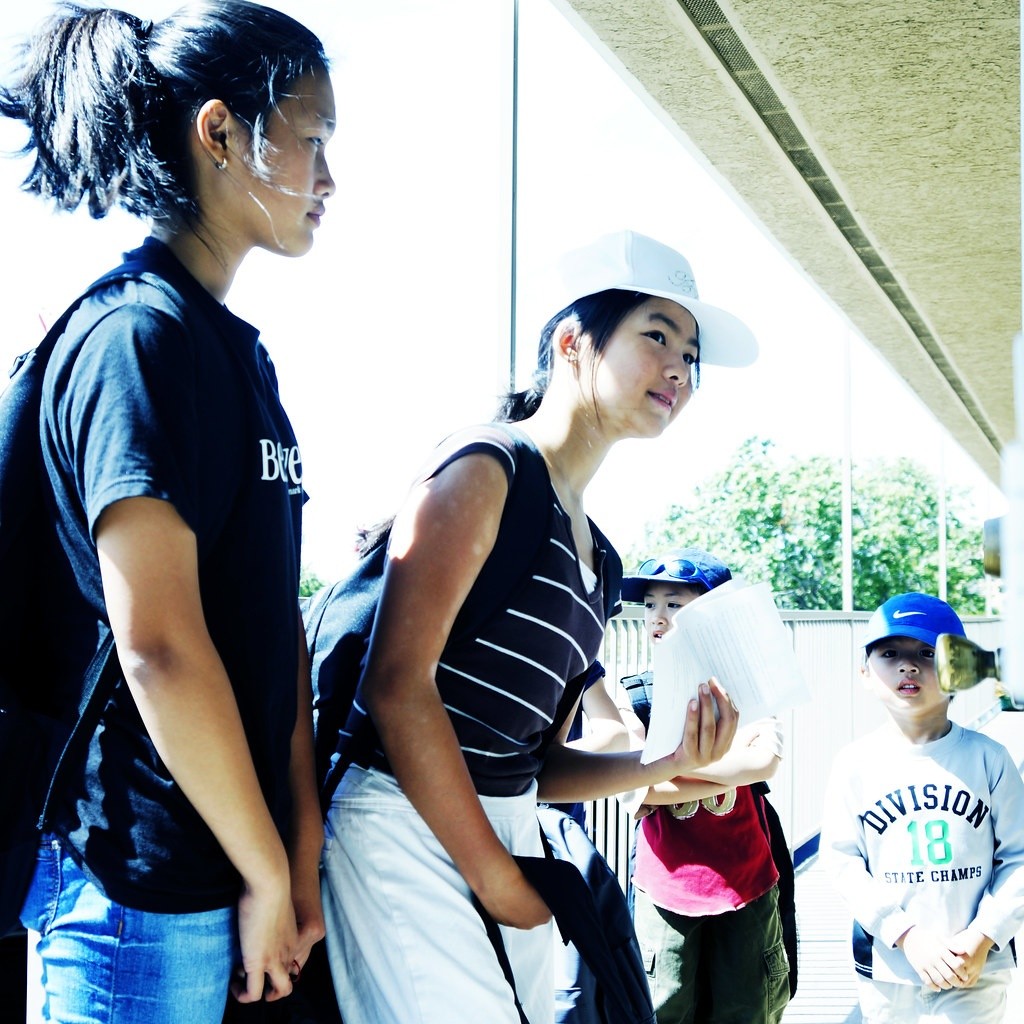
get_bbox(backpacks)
[0,266,288,939]
[299,423,623,822]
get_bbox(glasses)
[638,559,714,591]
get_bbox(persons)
[815,594,1024,1023]
[0,0,339,1024]
[620,548,799,1024]
[321,227,756,1024]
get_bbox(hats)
[534,234,701,372]
[864,592,967,657]
[619,548,733,603]
